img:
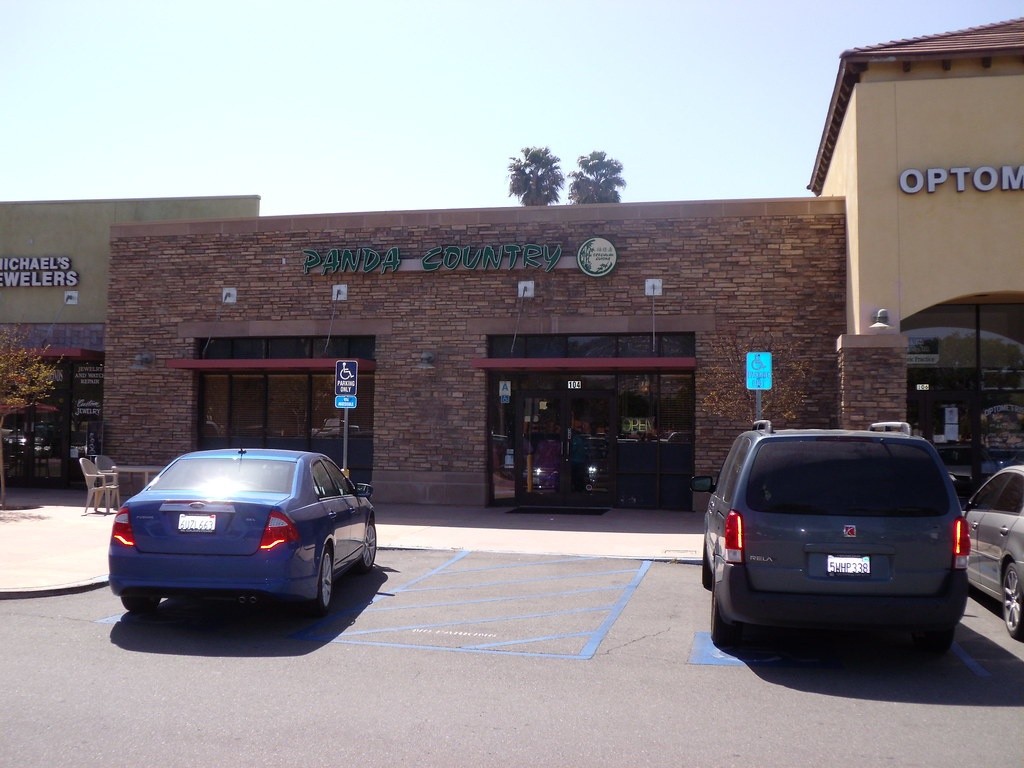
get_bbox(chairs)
[94,455,118,495]
[79,458,121,514]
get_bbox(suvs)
[685,419,973,656]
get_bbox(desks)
[110,464,168,505]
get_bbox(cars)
[959,464,1023,642]
[106,447,378,618]
[522,431,691,500]
[0,403,66,451]
[311,417,361,440]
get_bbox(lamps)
[869,308,895,329]
[412,352,437,370]
[129,351,154,370]
